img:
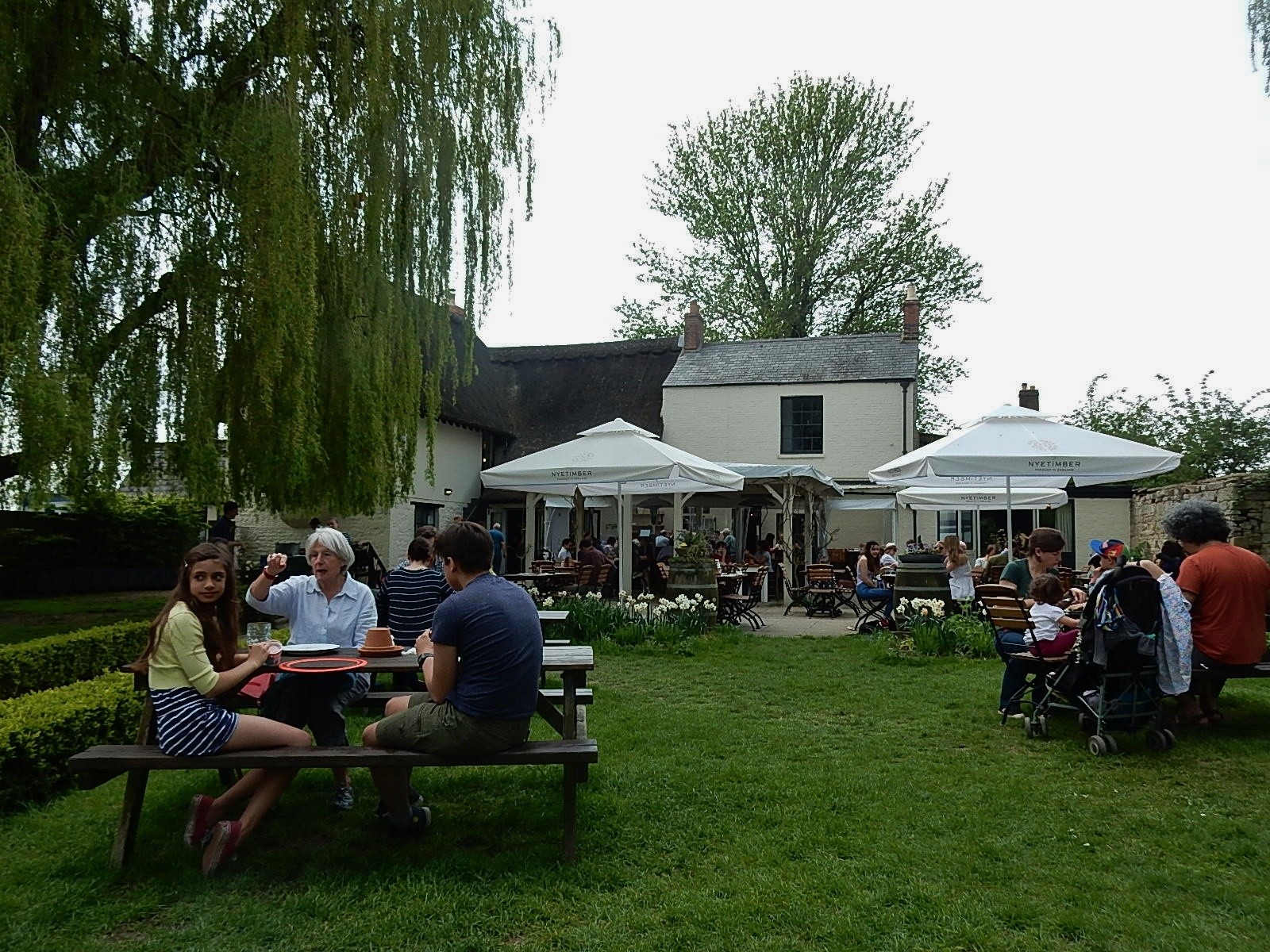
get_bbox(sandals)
[1165,709,1226,729]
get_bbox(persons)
[489,520,1016,629]
[1020,571,1082,658]
[208,500,239,557]
[1089,537,1131,596]
[359,519,544,840]
[310,517,322,530]
[240,526,378,812]
[374,537,457,646]
[1160,498,1270,726]
[394,525,446,576]
[327,516,354,546]
[142,541,315,883]
[991,526,1090,721]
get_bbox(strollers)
[1024,564,1181,757]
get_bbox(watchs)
[417,653,433,669]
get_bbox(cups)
[265,644,282,667]
[246,622,272,655]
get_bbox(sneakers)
[375,792,424,818]
[391,806,432,835]
[996,706,1024,719]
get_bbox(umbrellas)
[894,485,1068,560]
[867,403,1185,572]
[478,415,749,607]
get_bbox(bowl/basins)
[363,627,395,651]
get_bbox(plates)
[282,643,340,655]
[358,646,403,657]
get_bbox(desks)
[110,643,593,873]
[502,570,572,608]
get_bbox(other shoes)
[333,784,355,811]
[202,820,242,880]
[185,793,213,849]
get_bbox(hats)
[1090,538,1131,562]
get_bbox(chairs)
[972,584,1079,725]
[530,548,889,636]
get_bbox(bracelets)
[262,565,279,581]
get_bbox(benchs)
[63,737,601,774]
[353,689,597,707]
